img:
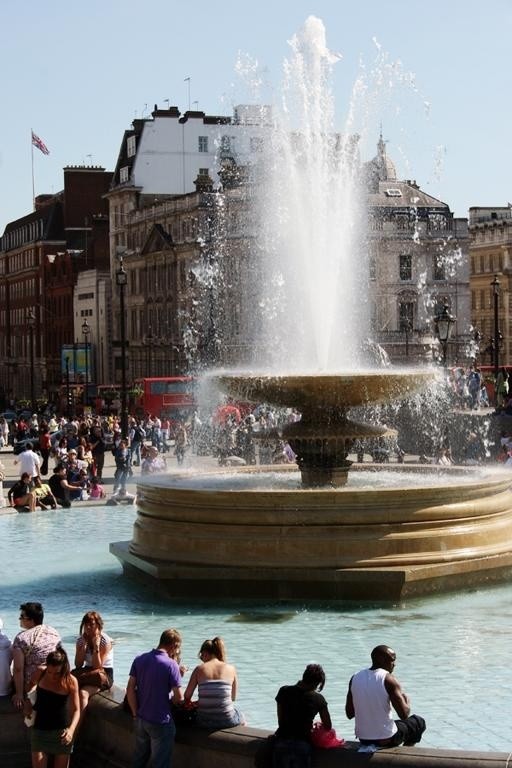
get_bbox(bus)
[479,365,510,407]
[56,384,130,416]
[134,376,260,431]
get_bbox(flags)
[31,133,49,156]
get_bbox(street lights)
[144,325,153,378]
[26,310,37,414]
[488,275,504,377]
[116,255,129,440]
[432,304,456,370]
[401,311,414,362]
[80,317,91,405]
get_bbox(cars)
[0,413,18,426]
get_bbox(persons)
[345,644,426,748]
[275,663,333,738]
[254,738,315,768]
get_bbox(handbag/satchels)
[313,720,346,750]
